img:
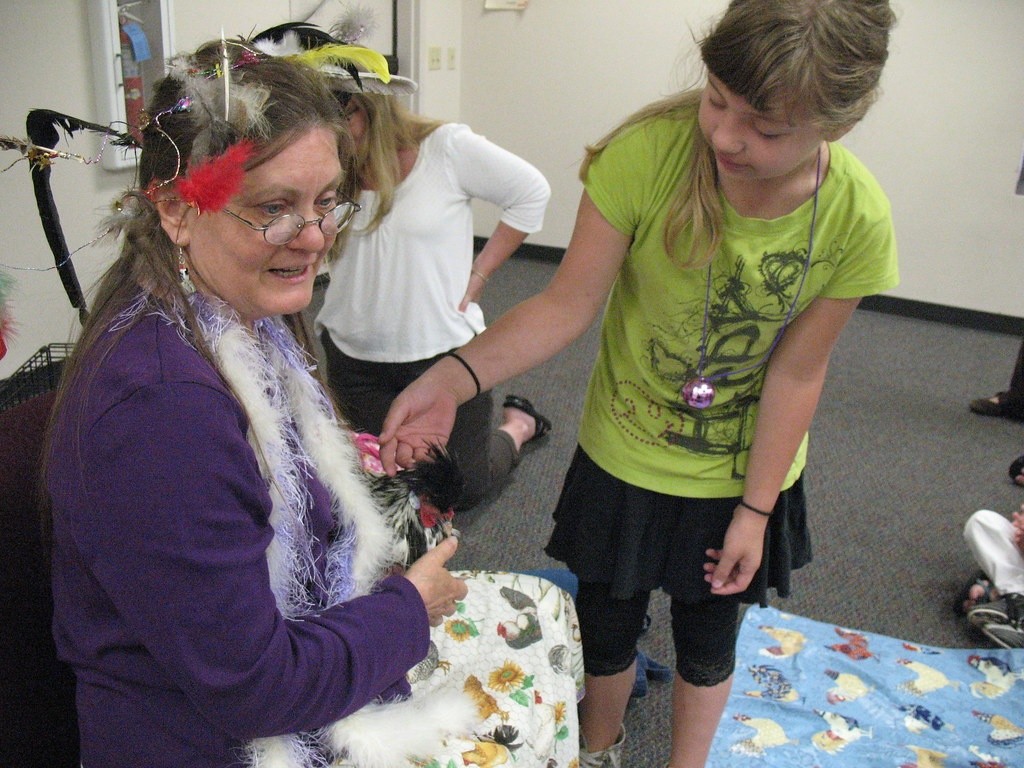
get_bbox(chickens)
[361,437,467,573]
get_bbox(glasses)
[218,188,363,245]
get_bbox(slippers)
[503,392,552,440]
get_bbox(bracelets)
[473,269,487,282]
[449,353,481,396]
[741,502,773,516]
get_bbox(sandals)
[953,568,1001,612]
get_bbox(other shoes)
[969,391,1007,418]
[1009,457,1024,486]
[982,622,1024,648]
[576,722,628,768]
[969,595,1024,626]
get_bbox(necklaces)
[683,152,821,408]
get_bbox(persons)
[378,0,900,768]
[954,505,1024,651]
[43,36,585,768]
[313,54,551,513]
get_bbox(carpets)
[704,601,1024,768]
